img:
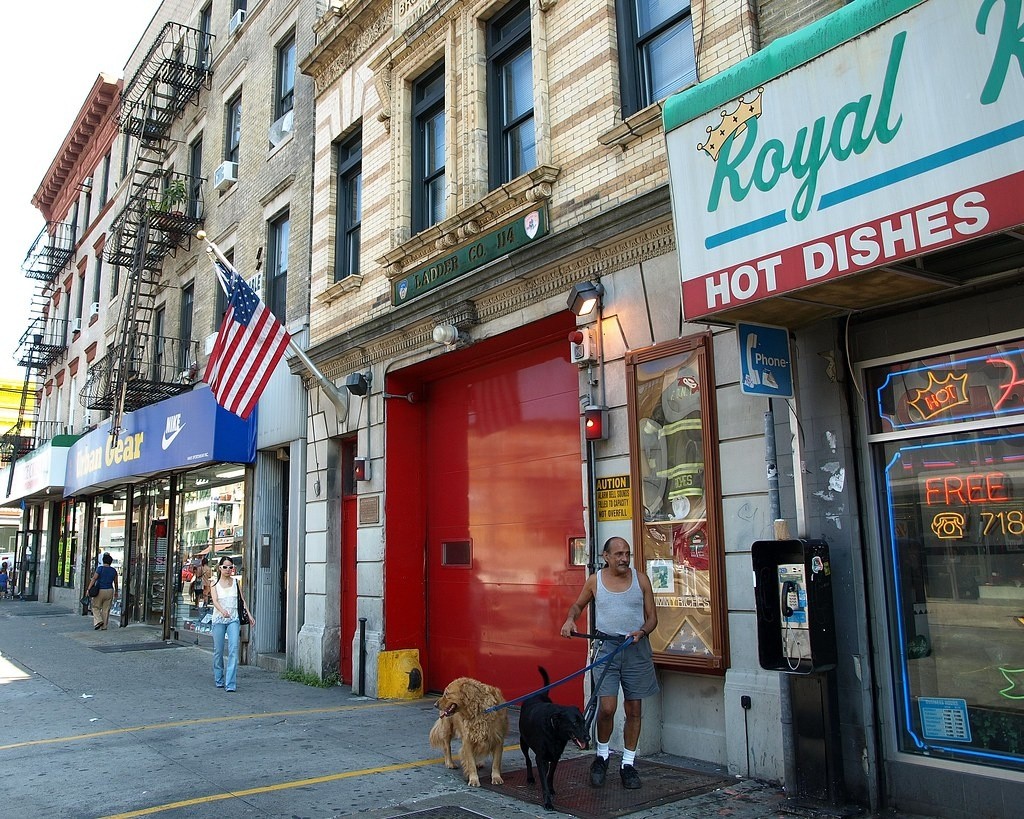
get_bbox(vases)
[168,227,182,243]
[33,334,43,344]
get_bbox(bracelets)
[640,628,648,636]
[87,588,90,590]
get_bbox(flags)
[201,248,293,417]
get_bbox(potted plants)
[146,200,170,226]
[163,180,188,223]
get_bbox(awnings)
[194,543,232,555]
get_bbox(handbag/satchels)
[235,579,251,624]
[194,579,204,592]
[80,596,89,606]
[89,586,99,597]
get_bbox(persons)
[85,555,118,630]
[561,537,659,791]
[0,557,13,601]
[98,549,110,566]
[210,557,255,694]
[189,559,212,612]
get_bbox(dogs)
[518,666,591,810]
[429,677,509,786]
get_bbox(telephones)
[780,577,799,617]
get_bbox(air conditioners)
[63,424,73,435]
[214,160,239,191]
[72,318,82,334]
[204,331,219,356]
[83,177,93,193]
[90,302,99,318]
[82,414,91,428]
[230,9,246,36]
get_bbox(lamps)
[346,373,368,397]
[46,487,55,494]
[566,282,604,316]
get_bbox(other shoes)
[191,607,198,611]
[94,622,103,630]
[226,688,234,691]
[216,682,222,687]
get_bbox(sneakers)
[589,753,610,786]
[618,763,642,789]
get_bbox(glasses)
[219,565,234,570]
